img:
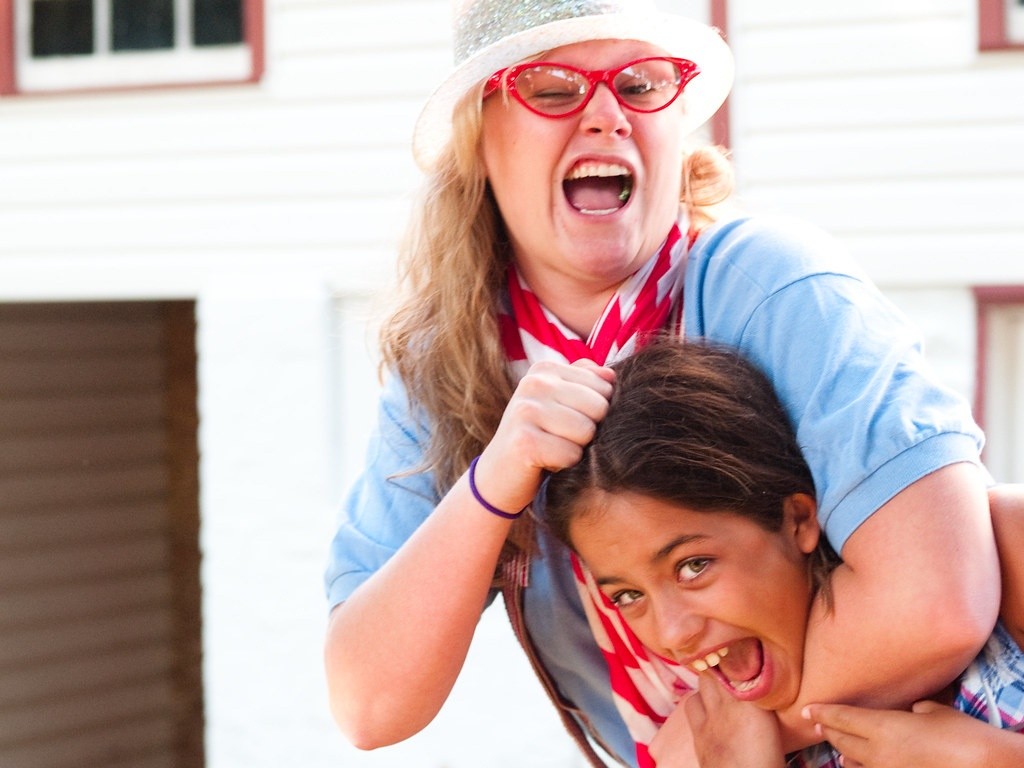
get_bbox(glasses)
[482,56,700,119]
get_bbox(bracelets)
[469,455,531,519]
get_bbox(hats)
[410,0,734,185]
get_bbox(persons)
[550,337,1024,768]
[320,1,1004,768]
